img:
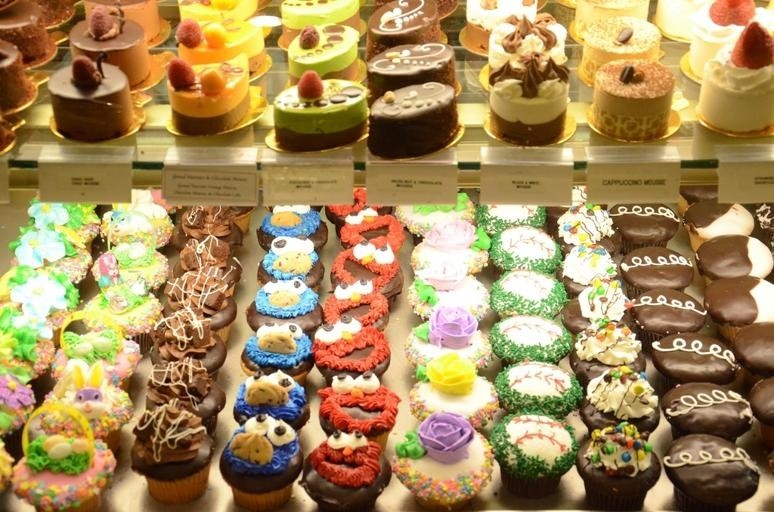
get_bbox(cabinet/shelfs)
[1,0,774,512]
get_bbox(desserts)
[0,0,774,161]
[1,185,774,512]
